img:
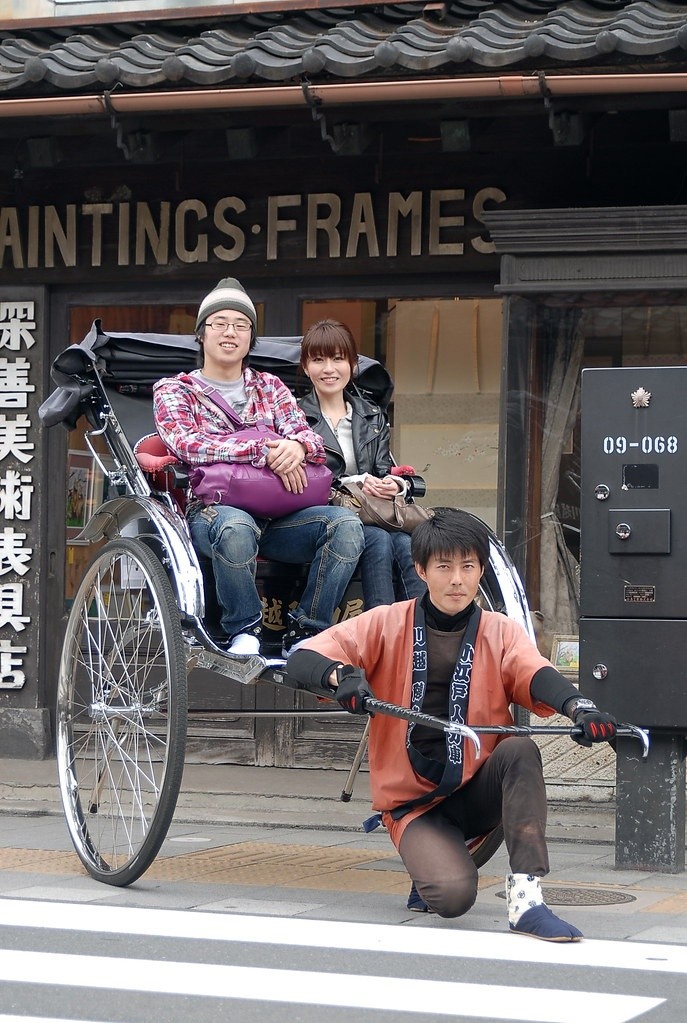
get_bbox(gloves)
[337,664,376,719]
[570,711,616,748]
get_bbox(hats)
[196,278,257,338]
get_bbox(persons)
[151,278,366,657]
[297,318,430,614]
[283,508,617,942]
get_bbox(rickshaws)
[37,328,651,889]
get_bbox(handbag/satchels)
[187,376,332,518]
[331,477,429,533]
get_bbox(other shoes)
[406,879,434,912]
[282,611,322,660]
[228,624,262,655]
[505,873,584,941]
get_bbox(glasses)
[202,321,252,331]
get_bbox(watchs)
[568,697,597,718]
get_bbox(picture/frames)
[549,635,580,668]
[97,585,133,619]
[65,449,125,547]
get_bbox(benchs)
[133,429,367,649]
